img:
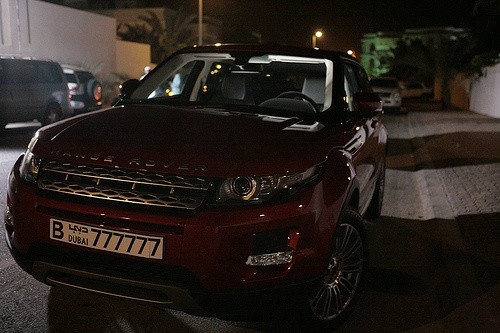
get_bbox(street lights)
[312,28,322,48]
[347,49,356,58]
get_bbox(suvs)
[5,42,388,326]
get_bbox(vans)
[61,63,102,115]
[370,77,402,111]
[1,55,70,130]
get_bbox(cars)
[399,80,433,102]
